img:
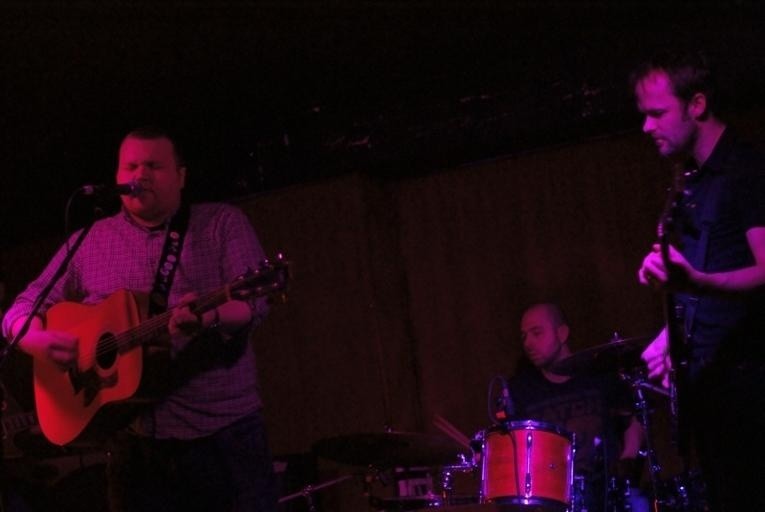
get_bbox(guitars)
[657,164,707,422]
[30,247,294,450]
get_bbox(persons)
[0,118,285,511]
[626,44,765,510]
[472,299,654,510]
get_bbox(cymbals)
[310,432,472,473]
[551,334,650,375]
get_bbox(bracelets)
[211,306,224,329]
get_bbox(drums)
[371,496,476,512]
[473,419,577,512]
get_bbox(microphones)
[82,180,142,198]
[501,383,515,416]
